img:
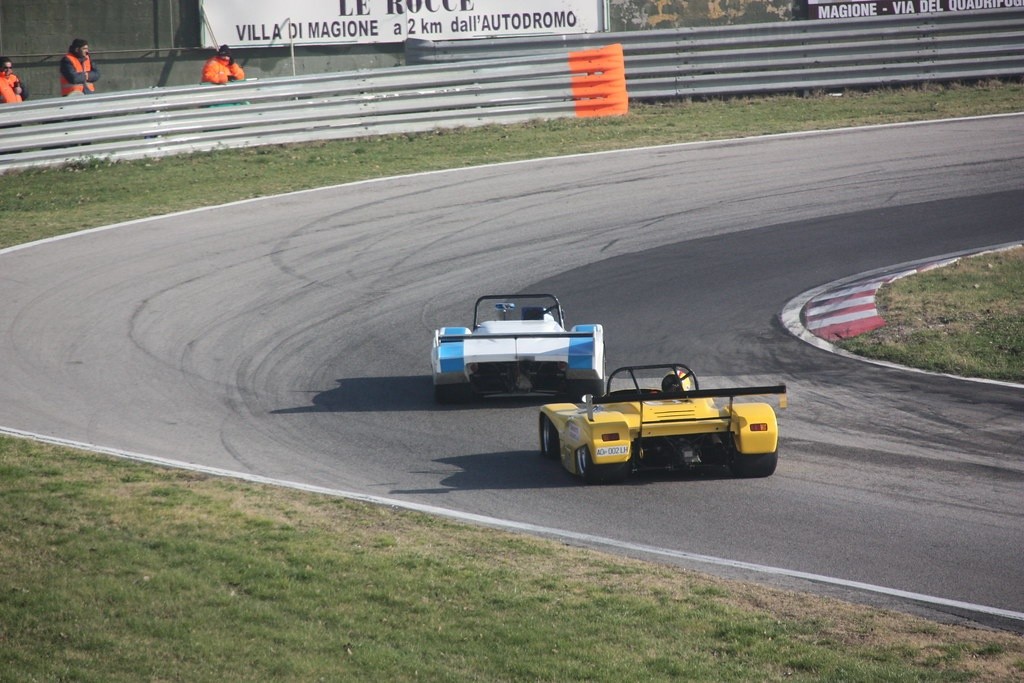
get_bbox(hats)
[73,38,88,47]
[219,45,231,56]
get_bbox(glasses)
[3,66,13,69]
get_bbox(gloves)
[227,74,238,83]
[227,53,235,65]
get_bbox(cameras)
[227,75,235,81]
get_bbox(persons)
[520,305,555,321]
[199,45,245,133]
[661,370,691,392]
[60,39,101,147]
[0,56,29,155]
